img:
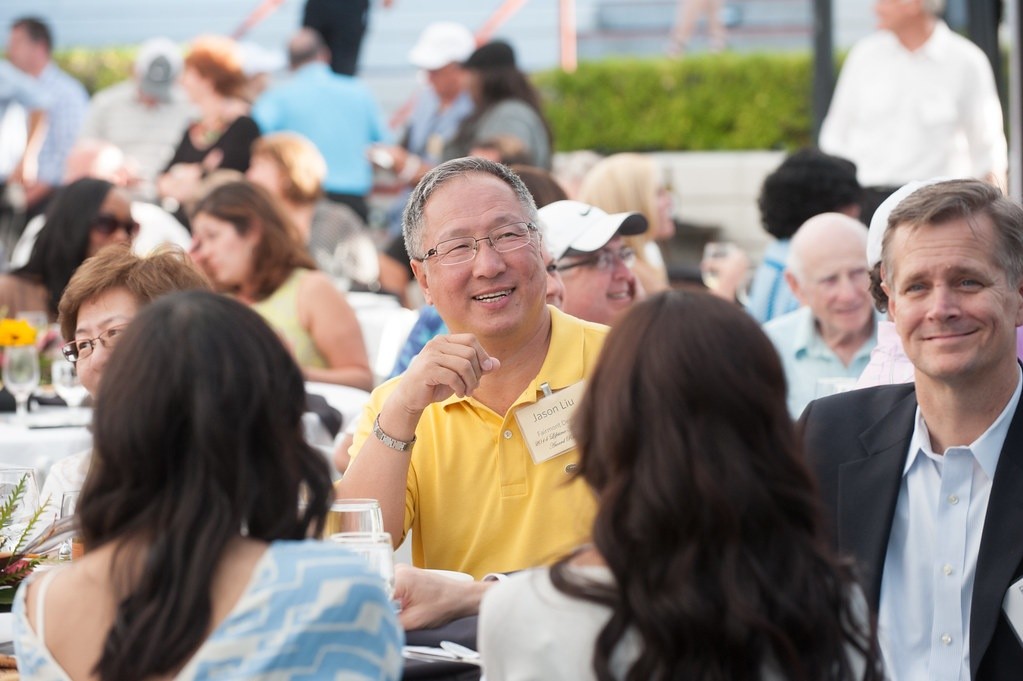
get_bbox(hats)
[413,27,475,73]
[136,45,181,102]
[536,200,648,268]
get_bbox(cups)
[701,242,738,293]
[321,498,396,607]
[1,468,79,562]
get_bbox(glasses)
[548,246,635,272]
[420,221,537,266]
[63,321,132,363]
[96,218,140,240]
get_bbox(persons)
[818,0,1009,223]
[389,178,1023,681]
[190,179,371,392]
[747,152,862,321]
[328,157,605,573]
[35,246,213,514]
[11,291,402,681]
[478,288,884,681]
[761,212,886,421]
[1,19,556,323]
[386,154,748,381]
[854,178,1023,386]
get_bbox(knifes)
[440,641,478,662]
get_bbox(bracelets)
[373,413,417,451]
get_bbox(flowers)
[0,316,66,385]
[0,472,56,587]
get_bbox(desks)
[0,381,369,588]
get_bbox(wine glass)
[4,345,90,431]
